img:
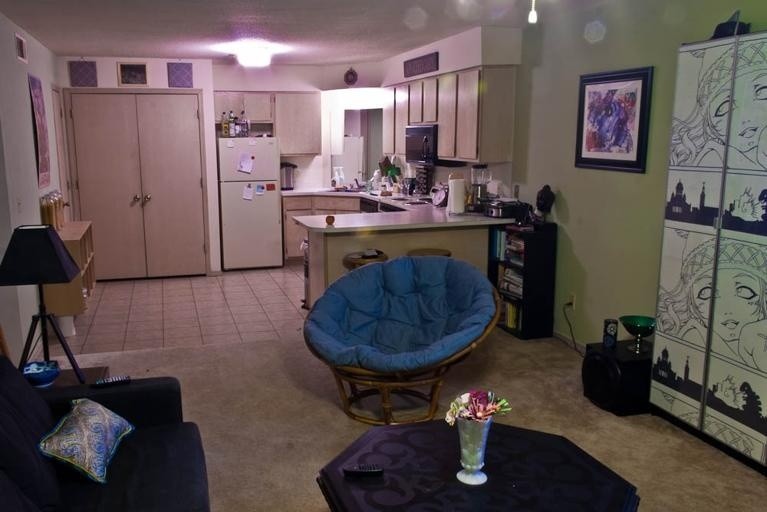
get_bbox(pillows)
[39,396,134,486]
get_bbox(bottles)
[229,111,235,137]
[235,117,241,136]
[604,319,618,352]
[222,113,229,137]
[240,111,248,137]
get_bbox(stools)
[337,251,391,271]
[401,245,451,258]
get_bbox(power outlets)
[566,292,578,310]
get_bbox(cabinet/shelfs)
[649,34,767,477]
[409,82,422,161]
[62,84,214,282]
[493,219,557,339]
[291,185,517,313]
[273,92,323,156]
[394,83,410,156]
[43,218,98,337]
[436,73,458,159]
[421,77,438,161]
[458,70,512,164]
[215,90,272,124]
[380,84,396,158]
[281,189,361,263]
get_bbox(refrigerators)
[217,136,284,271]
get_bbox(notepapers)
[237,151,254,174]
[255,183,265,195]
[242,183,254,201]
[227,140,234,148]
[248,138,258,146]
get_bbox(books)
[500,268,523,296]
[497,301,521,328]
[497,230,524,266]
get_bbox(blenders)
[469,164,492,212]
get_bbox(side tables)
[53,364,114,389]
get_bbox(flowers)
[443,385,514,426]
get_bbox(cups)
[373,174,382,192]
[448,179,465,214]
[400,178,409,199]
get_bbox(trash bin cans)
[301,237,309,278]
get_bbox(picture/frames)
[14,31,30,62]
[575,66,653,173]
[115,60,152,84]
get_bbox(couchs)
[0,342,211,512]
[303,255,503,428]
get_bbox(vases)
[455,416,493,486]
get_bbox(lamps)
[208,36,294,70]
[0,227,86,386]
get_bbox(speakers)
[603,317,618,348]
[582,339,654,418]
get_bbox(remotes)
[95,376,132,386]
[343,463,384,475]
